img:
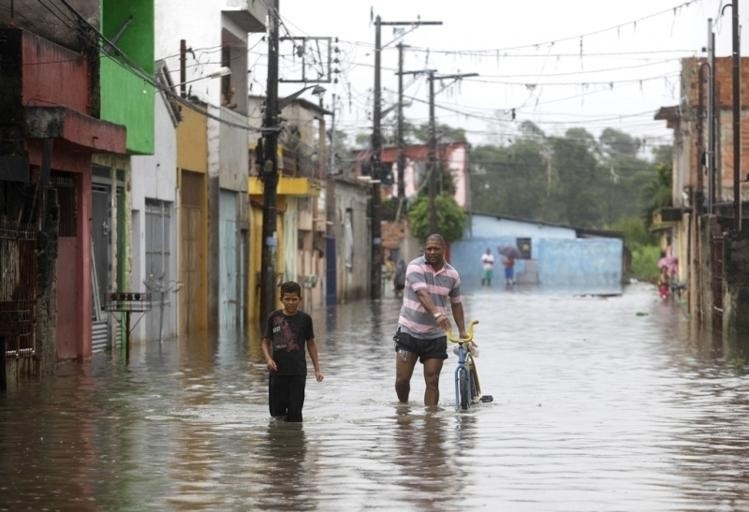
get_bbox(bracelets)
[432,311,443,321]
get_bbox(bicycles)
[446,319,492,409]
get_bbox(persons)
[260,281,325,424]
[390,230,480,411]
[392,257,406,299]
[668,258,681,300]
[502,256,517,287]
[480,246,496,286]
[656,266,670,299]
[656,250,670,277]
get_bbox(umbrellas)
[497,241,522,261]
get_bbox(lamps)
[278,84,326,115]
[153,67,232,96]
